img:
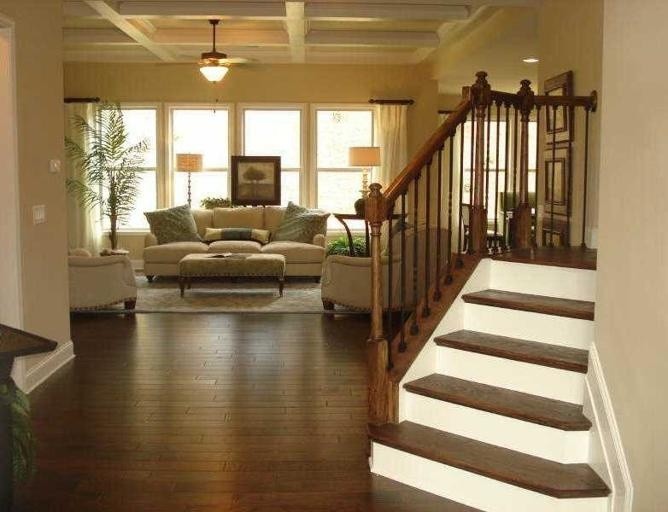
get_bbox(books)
[204,252,251,258]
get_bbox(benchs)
[178,252,284,297]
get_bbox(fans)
[174,19,258,65]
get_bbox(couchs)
[145,207,328,282]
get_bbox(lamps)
[349,147,381,197]
[200,65,229,84]
[176,152,203,206]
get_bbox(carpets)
[89,275,356,313]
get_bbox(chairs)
[322,222,451,318]
[460,203,503,252]
[68,246,137,314]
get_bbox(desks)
[333,213,408,256]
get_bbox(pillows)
[143,201,331,243]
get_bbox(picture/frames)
[232,155,281,205]
